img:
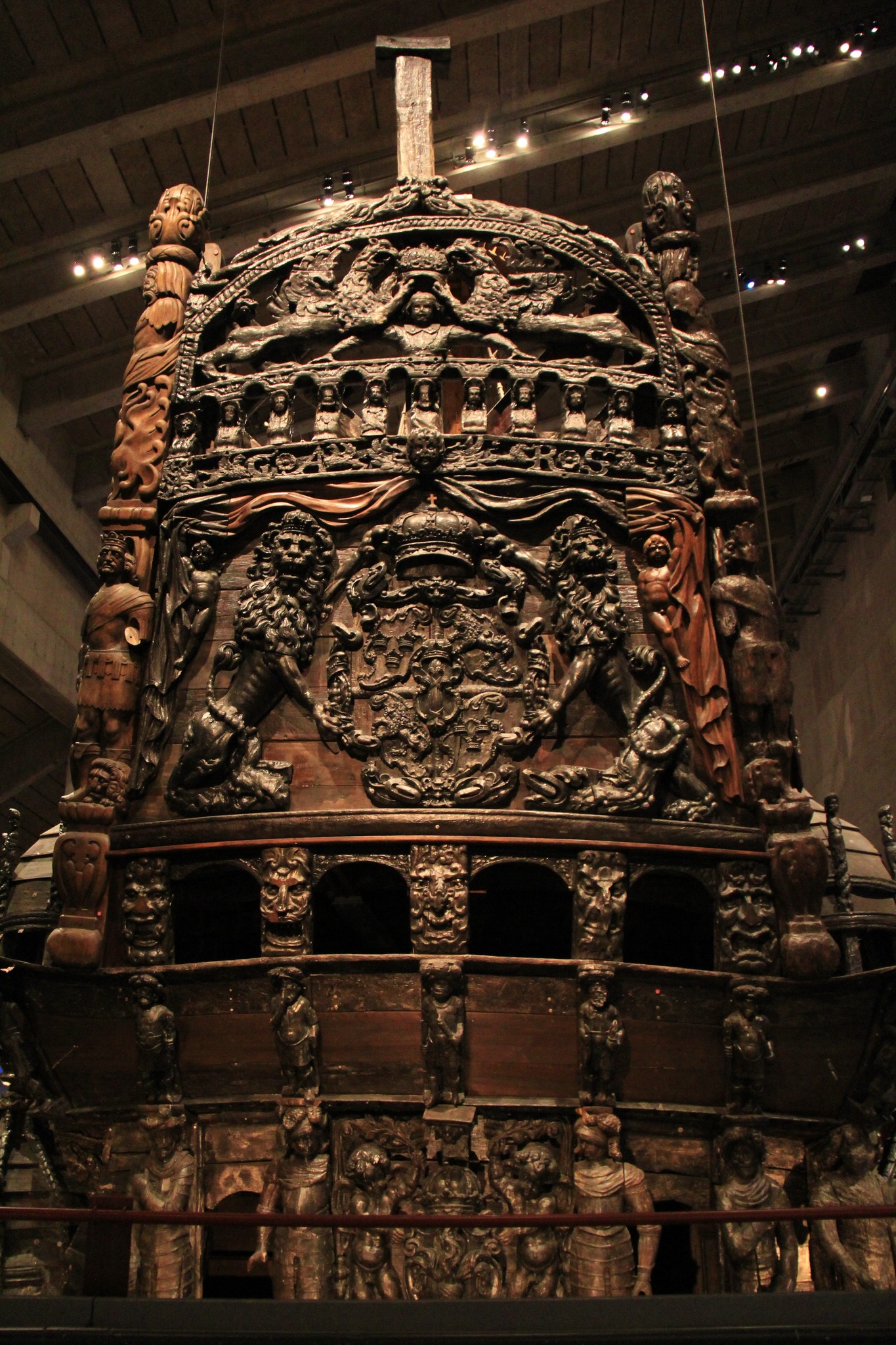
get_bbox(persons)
[126,1104,197,1300]
[60,529,154,804]
[268,966,319,1094]
[577,981,624,1104]
[245,1101,333,1300]
[716,1126,799,1294]
[637,517,693,668]
[724,997,775,1114]
[419,977,465,1107]
[807,1123,896,1291]
[128,973,181,1098]
[569,1106,662,1300]
